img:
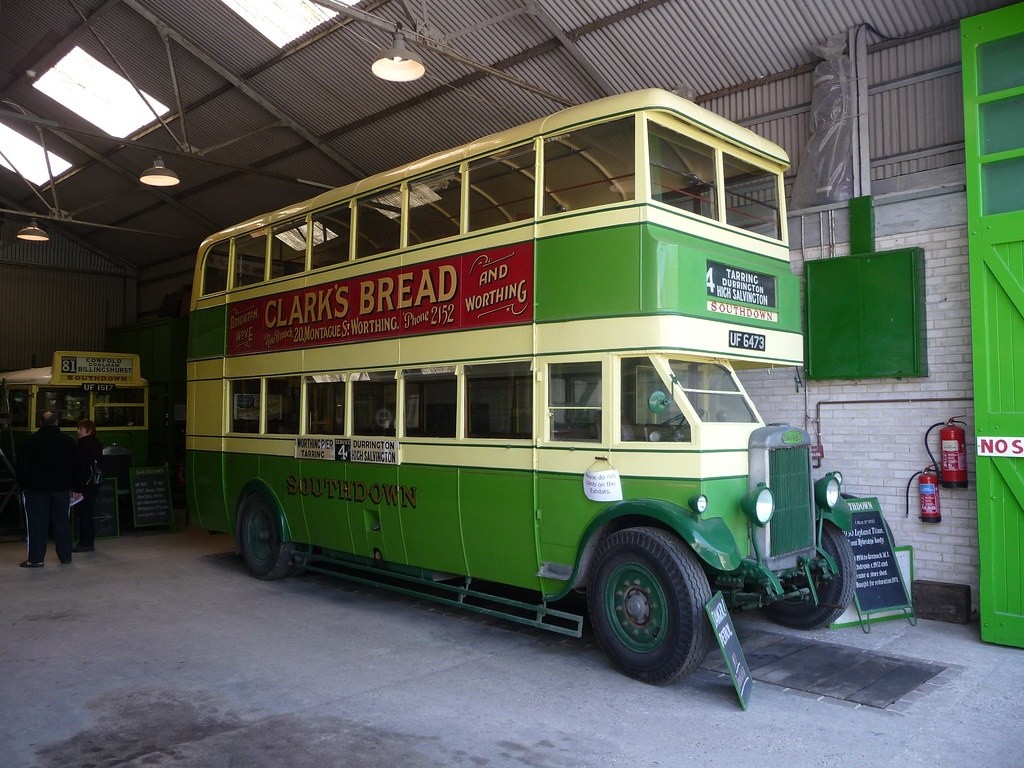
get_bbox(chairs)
[593,411,635,441]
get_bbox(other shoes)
[20,561,45,568]
[60,558,71,564]
[72,545,96,553]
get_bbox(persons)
[14,411,82,568]
[72,418,103,552]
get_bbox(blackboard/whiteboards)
[835,496,914,615]
[70,477,120,543]
[128,466,175,528]
[825,544,914,630]
[705,588,755,712]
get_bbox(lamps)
[368,34,424,83]
[139,157,180,187]
[17,218,50,241]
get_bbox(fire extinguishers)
[923,414,969,489]
[902,461,942,523]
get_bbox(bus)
[0,350,149,494]
[185,88,857,686]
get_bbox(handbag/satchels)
[85,464,104,486]
